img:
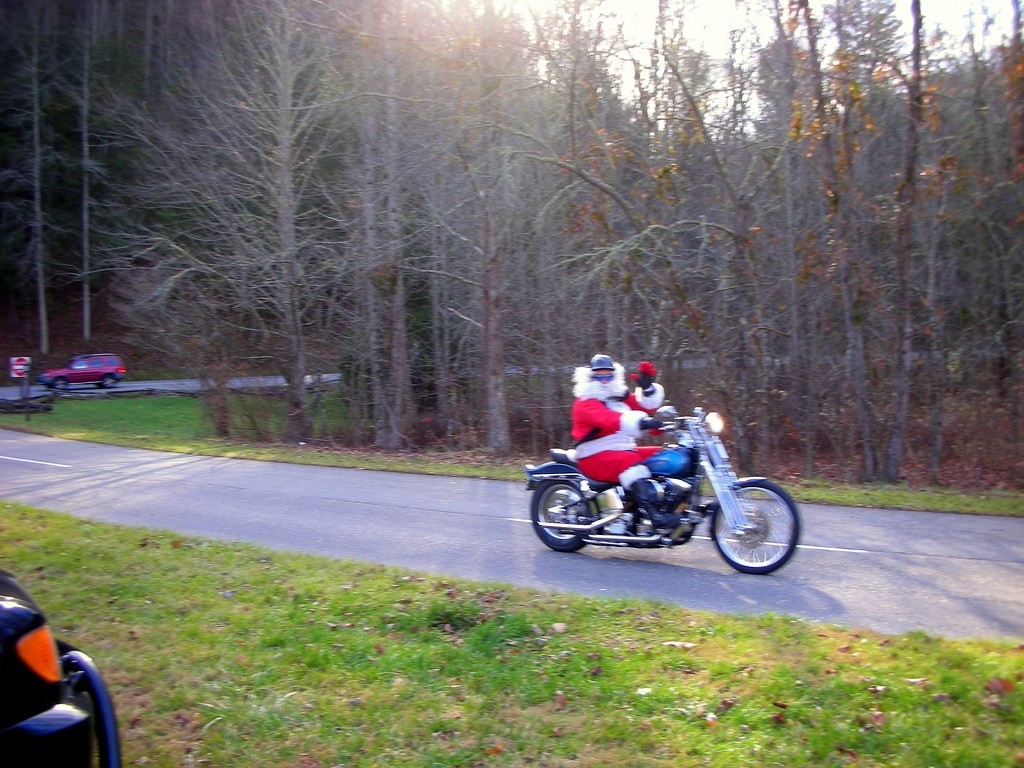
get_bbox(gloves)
[629,361,658,392]
[639,417,666,436]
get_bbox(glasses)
[593,374,614,380]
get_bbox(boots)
[631,478,681,529]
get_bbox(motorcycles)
[524,405,801,575]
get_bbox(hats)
[591,354,615,372]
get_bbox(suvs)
[0,570,122,768]
[33,352,128,390]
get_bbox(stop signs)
[12,357,30,377]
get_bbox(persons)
[567,355,684,526]
